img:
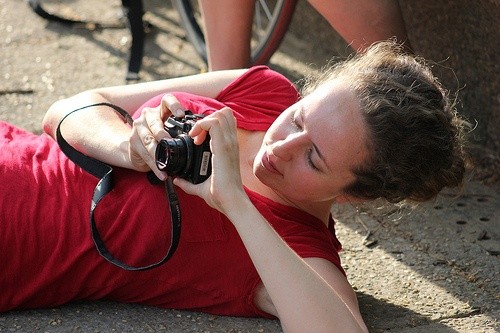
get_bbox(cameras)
[155,110,212,184]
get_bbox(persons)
[0,37,473,333]
[200,1,415,72]
